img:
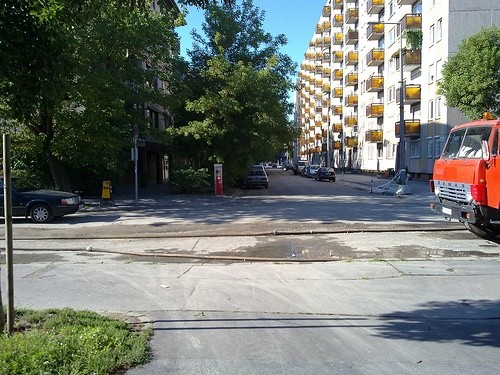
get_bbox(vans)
[308,165,321,177]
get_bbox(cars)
[253,164,266,170]
[245,169,268,190]
[272,163,277,169]
[314,167,335,182]
[285,164,294,171]
[0,176,83,223]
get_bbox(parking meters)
[214,163,223,196]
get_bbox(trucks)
[430,113,500,236]
[291,160,308,175]
